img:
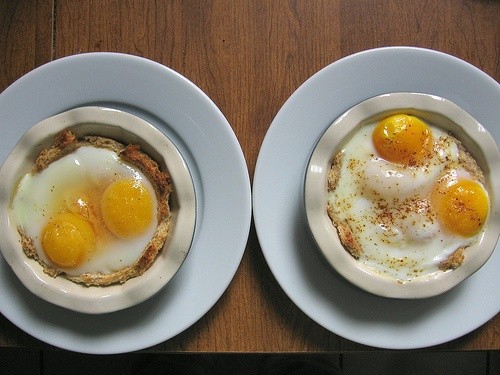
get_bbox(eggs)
[11,145,157,277]
[327,113,491,277]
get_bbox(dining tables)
[0,0,500,354]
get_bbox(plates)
[252,43,500,350]
[0,52,251,355]
[304,91,500,299]
[0,103,199,317]
